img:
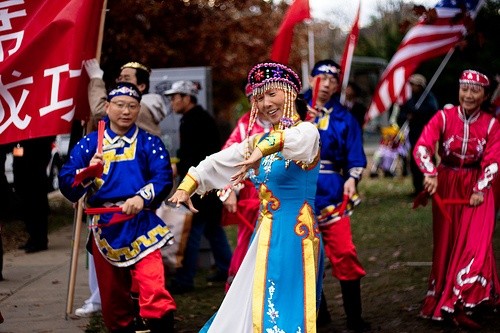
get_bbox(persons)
[162,79,232,280]
[75,59,168,316]
[13,134,57,256]
[405,74,439,198]
[302,59,370,333]
[167,62,325,333]
[412,67,500,329]
[58,82,177,333]
[342,82,368,126]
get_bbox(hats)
[164,80,197,96]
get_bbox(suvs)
[348,58,390,105]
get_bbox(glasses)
[109,100,138,111]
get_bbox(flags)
[270,0,311,64]
[365,0,482,121]
[337,12,360,91]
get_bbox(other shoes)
[19,240,47,252]
[206,273,229,282]
[75,303,101,317]
[168,284,193,293]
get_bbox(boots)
[317,290,331,328]
[147,311,174,333]
[341,280,371,331]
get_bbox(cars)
[5,132,70,191]
